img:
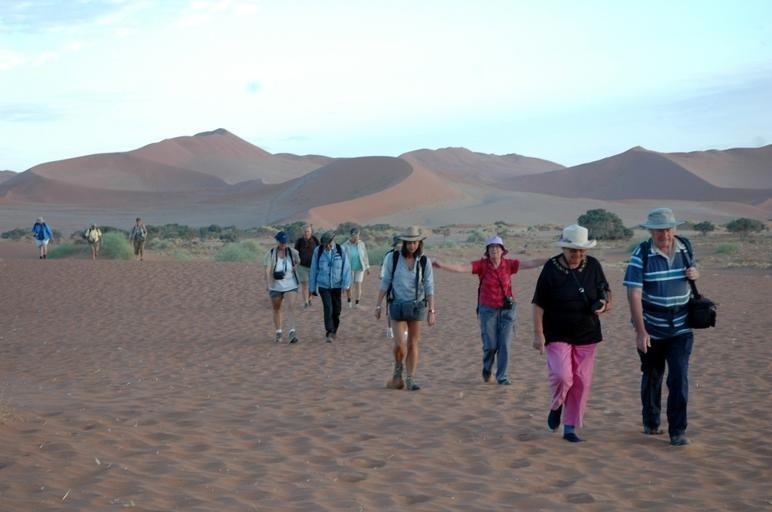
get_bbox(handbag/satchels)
[687,293,718,330]
[389,297,423,323]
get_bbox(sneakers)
[482,367,491,382]
[547,404,563,432]
[671,433,689,445]
[497,377,511,385]
[406,378,421,391]
[644,421,665,434]
[564,432,579,443]
[387,327,393,339]
[325,300,361,343]
[393,362,404,381]
[275,298,314,344]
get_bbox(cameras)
[502,295,515,309]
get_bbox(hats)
[550,222,597,251]
[275,231,288,243]
[391,236,403,247]
[397,225,427,242]
[639,208,686,230]
[35,217,45,223]
[483,234,509,257]
[320,231,336,246]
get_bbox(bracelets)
[375,306,381,309]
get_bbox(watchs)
[429,310,436,314]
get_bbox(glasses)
[569,249,584,253]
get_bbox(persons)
[374,225,436,390]
[379,234,407,340]
[432,237,553,384]
[85,223,102,260]
[265,225,371,343]
[129,217,147,261]
[531,225,612,442]
[32,217,53,258]
[623,208,699,445]
